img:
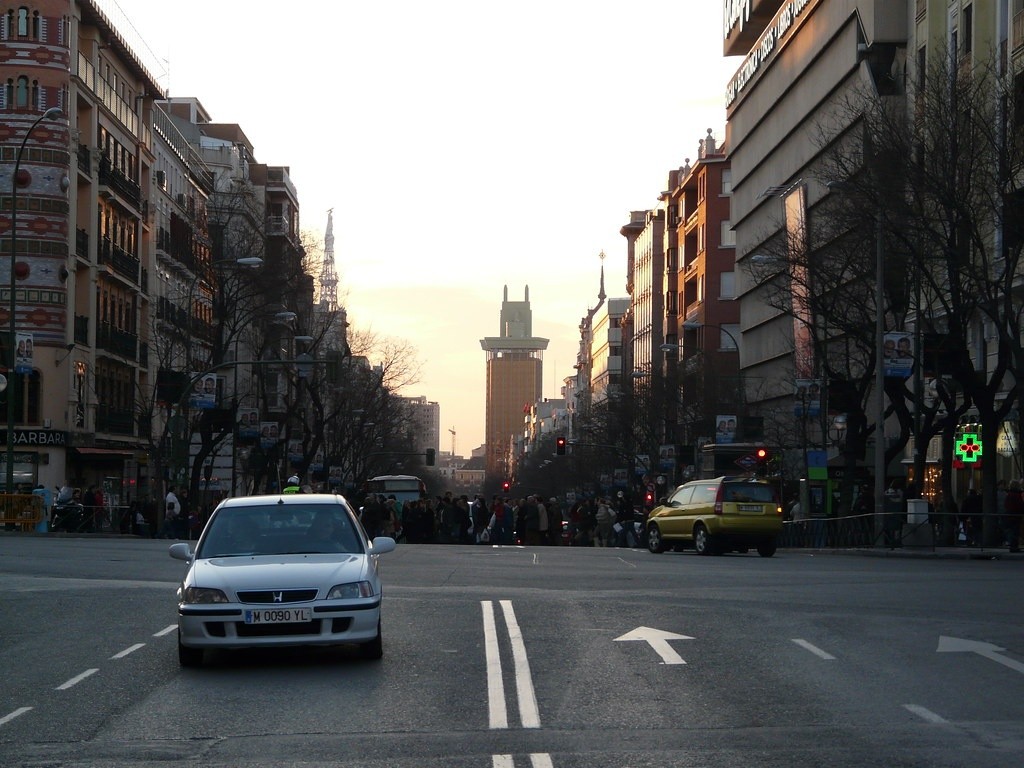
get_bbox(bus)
[366,475,426,509]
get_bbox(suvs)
[645,475,783,553]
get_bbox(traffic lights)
[503,482,510,493]
[755,449,766,469]
[556,437,566,455]
[645,485,654,502]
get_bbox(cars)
[627,512,644,547]
[170,493,396,668]
[440,501,474,534]
[559,519,571,543]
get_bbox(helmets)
[288,475,300,486]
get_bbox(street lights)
[630,372,681,418]
[7,108,64,530]
[256,336,313,410]
[681,322,742,403]
[658,343,716,403]
[231,312,297,495]
[183,257,263,496]
[752,255,836,452]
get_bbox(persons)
[193,378,215,394]
[796,383,820,401]
[307,510,345,550]
[661,448,674,459]
[998,478,1024,551]
[717,420,735,432]
[935,487,983,545]
[239,412,257,426]
[615,472,626,479]
[336,488,640,547]
[636,458,648,467]
[699,441,711,453]
[782,492,807,545]
[330,469,340,477]
[284,476,305,494]
[224,515,265,553]
[13,482,207,540]
[289,443,302,454]
[883,337,912,359]
[15,338,33,356]
[303,485,313,494]
[312,455,321,464]
[259,425,277,437]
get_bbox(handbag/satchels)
[480,529,490,542]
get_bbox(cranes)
[449,425,456,463]
